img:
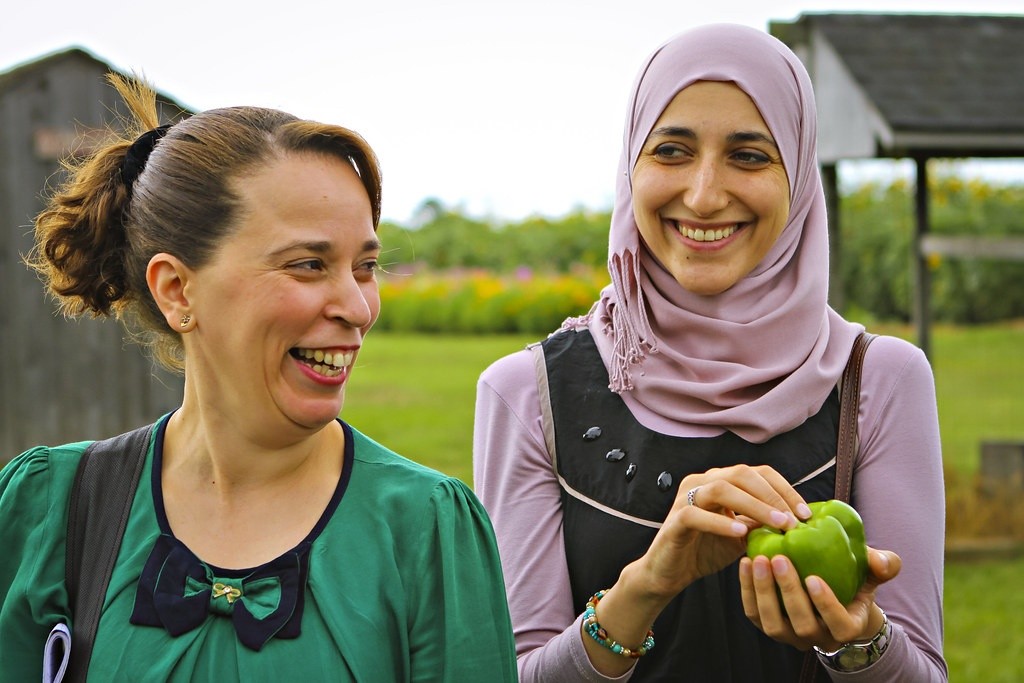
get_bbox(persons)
[0,69,517,683]
[470,24,951,683]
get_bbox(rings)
[687,486,702,505]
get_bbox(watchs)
[812,612,892,673]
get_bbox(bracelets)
[583,588,656,659]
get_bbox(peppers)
[742,499,869,621]
